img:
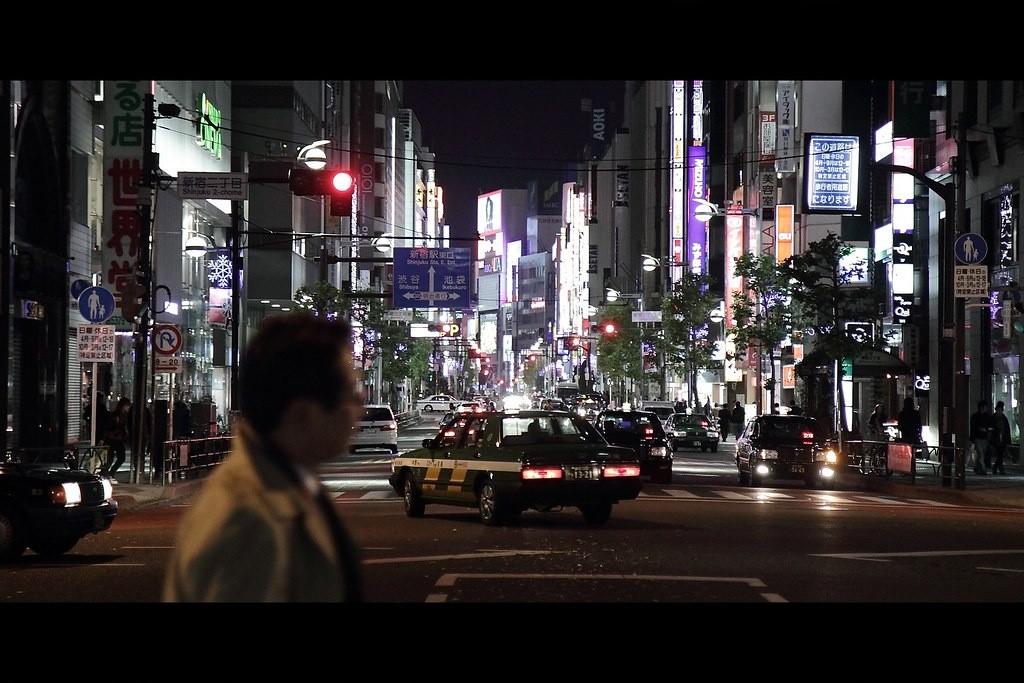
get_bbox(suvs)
[348,405,398,454]
[0,462,118,561]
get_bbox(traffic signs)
[393,247,471,308]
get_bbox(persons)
[654,396,702,413]
[711,401,744,441]
[774,399,804,416]
[82,392,108,440]
[868,404,887,466]
[899,397,922,470]
[159,316,370,601]
[970,401,1010,474]
[102,398,131,484]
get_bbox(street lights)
[641,254,692,407]
[606,287,645,400]
[692,198,762,415]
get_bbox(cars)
[389,411,642,525]
[530,393,720,483]
[416,394,505,442]
[734,414,839,489]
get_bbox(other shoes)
[109,476,118,485]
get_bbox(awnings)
[841,349,910,381]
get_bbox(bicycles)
[859,433,894,477]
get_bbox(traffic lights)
[428,324,451,332]
[591,324,615,333]
[289,170,353,216]
